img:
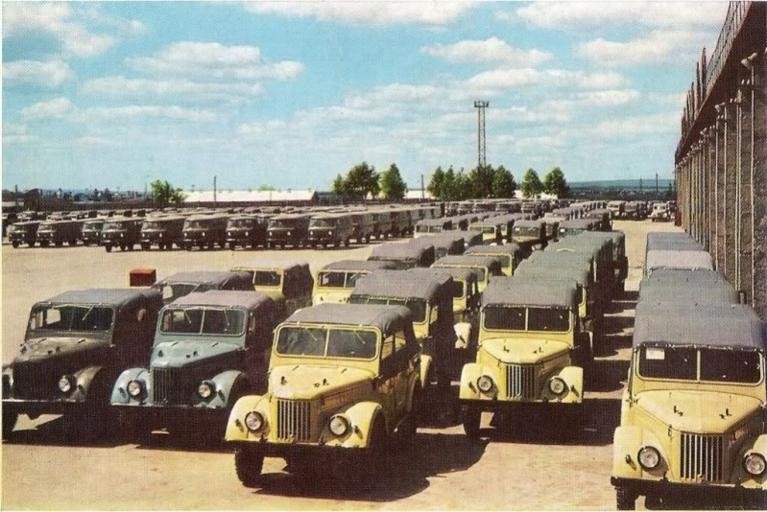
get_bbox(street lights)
[473,98,491,174]
